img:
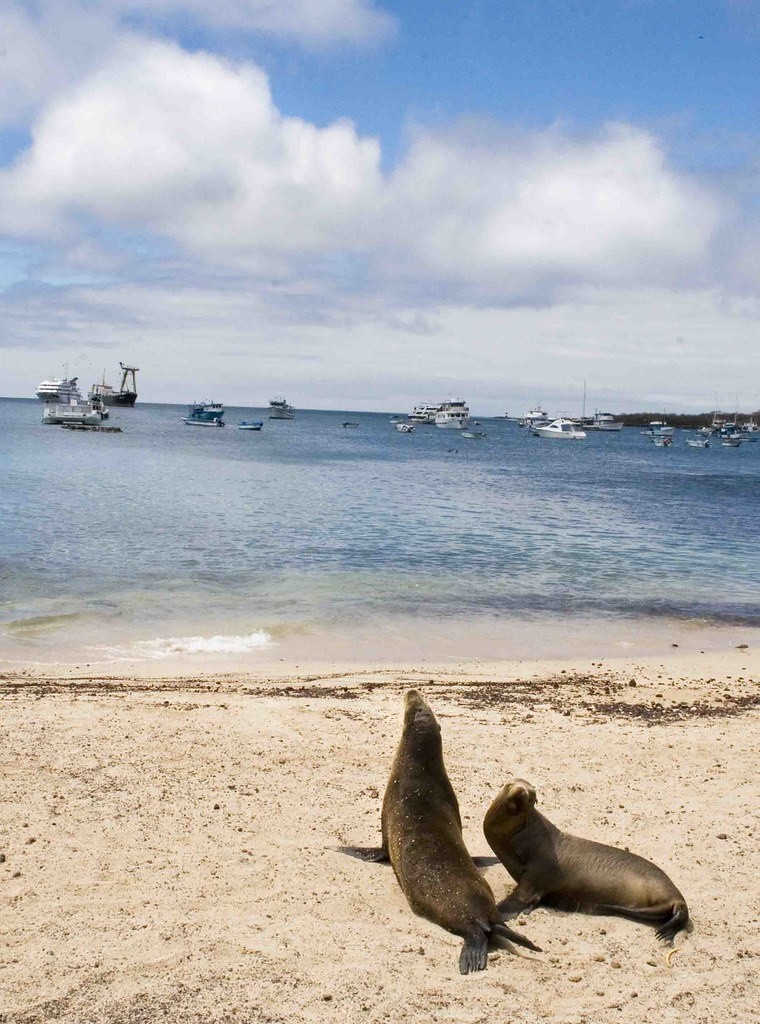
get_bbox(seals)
[323,689,544,975]
[483,778,688,941]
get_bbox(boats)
[640,421,675,436]
[61,420,123,432]
[339,422,360,428]
[394,423,416,433]
[533,418,587,440]
[435,396,471,429]
[685,438,712,448]
[268,395,297,419]
[721,438,743,447]
[461,432,488,439]
[581,410,628,431]
[652,435,675,447]
[570,417,594,427]
[696,407,760,442]
[237,418,264,430]
[87,361,140,407]
[181,398,225,427]
[42,402,102,427]
[517,406,551,430]
[407,399,440,425]
[35,362,84,404]
